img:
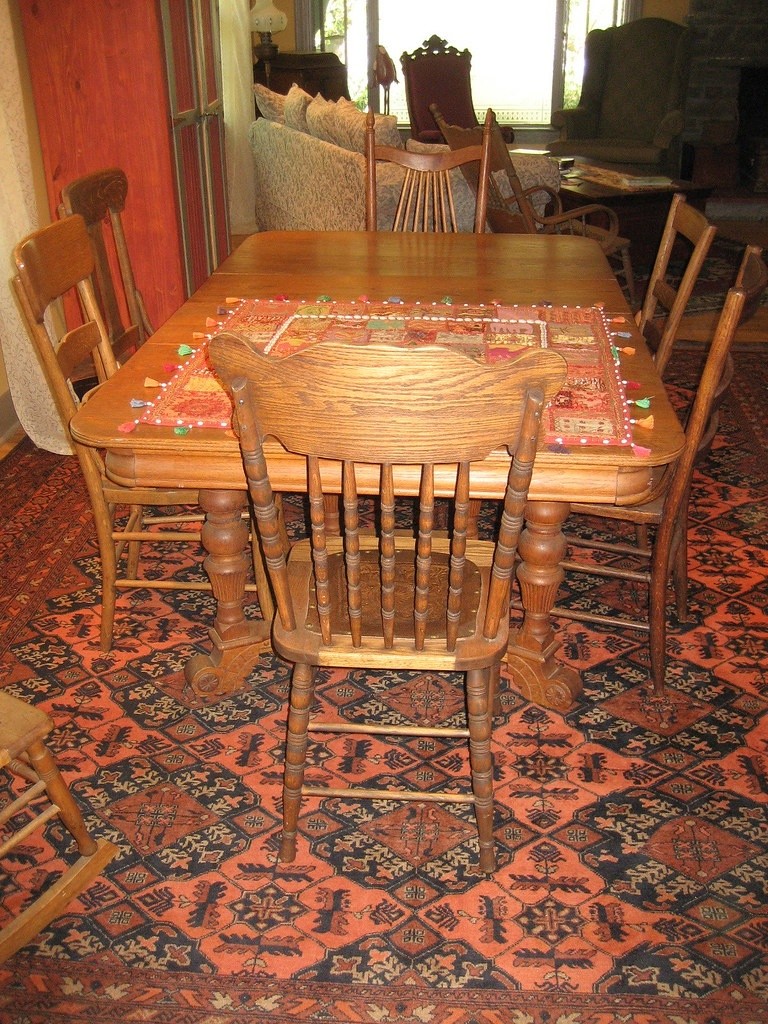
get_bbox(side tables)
[255,48,352,120]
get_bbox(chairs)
[634,193,719,377]
[429,103,636,308]
[401,34,515,145]
[206,334,568,873]
[55,168,155,371]
[508,244,768,695]
[6,213,286,652]
[0,689,121,969]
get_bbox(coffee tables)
[546,154,716,282]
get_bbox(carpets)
[550,223,768,319]
[0,340,768,1024]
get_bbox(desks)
[69,231,686,716]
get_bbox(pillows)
[252,83,404,163]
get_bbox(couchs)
[250,81,559,240]
[543,18,694,181]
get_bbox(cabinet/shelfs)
[18,0,232,373]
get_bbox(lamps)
[248,0,287,60]
